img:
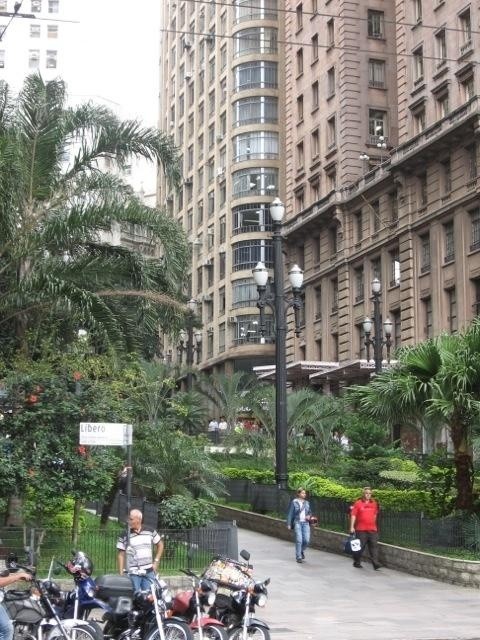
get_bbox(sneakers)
[302,552,305,559]
[297,559,302,563]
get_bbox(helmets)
[67,551,94,576]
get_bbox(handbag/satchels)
[202,554,256,590]
[345,531,363,556]
[290,517,295,530]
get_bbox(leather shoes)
[374,563,383,570]
[354,562,363,568]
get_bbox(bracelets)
[155,559,160,565]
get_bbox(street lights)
[363,275,397,374]
[251,196,304,492]
[180,299,203,392]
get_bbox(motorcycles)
[0,543,230,640]
[203,550,272,639]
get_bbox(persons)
[0,571,33,640]
[116,508,164,592]
[197,413,350,458]
[286,487,318,563]
[344,487,386,570]
[117,463,132,497]
